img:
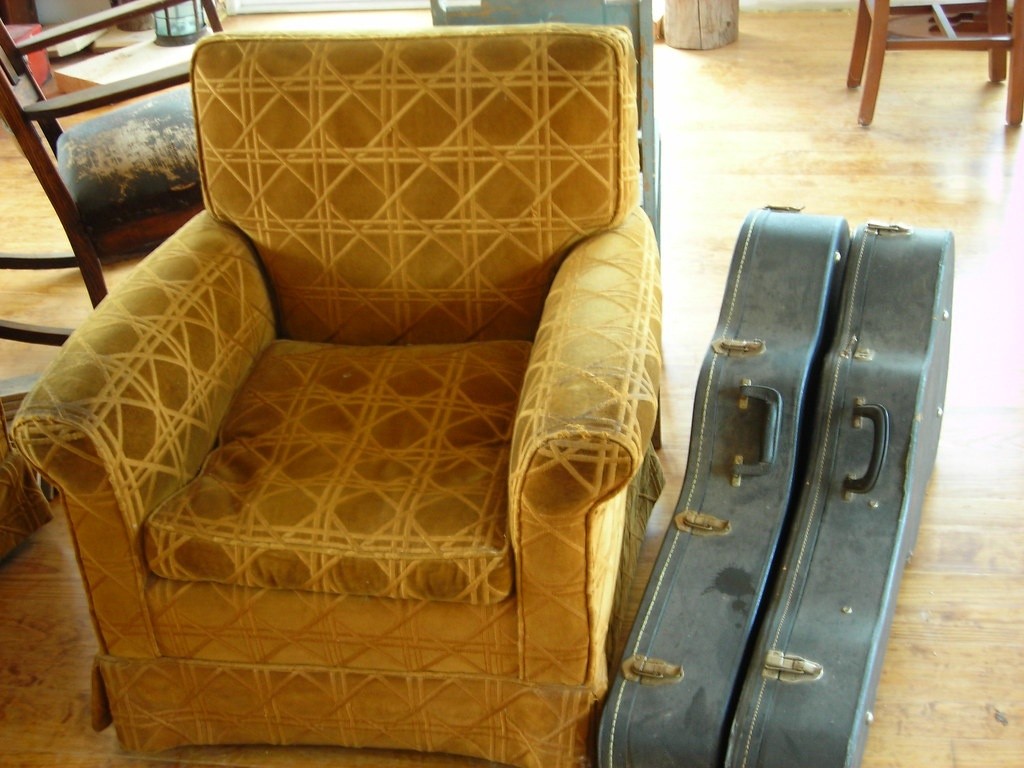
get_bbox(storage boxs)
[0,22,52,119]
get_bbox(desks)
[54,27,215,114]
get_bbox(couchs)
[8,20,664,768]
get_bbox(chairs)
[847,0,1024,129]
[428,0,662,250]
[0,0,224,309]
